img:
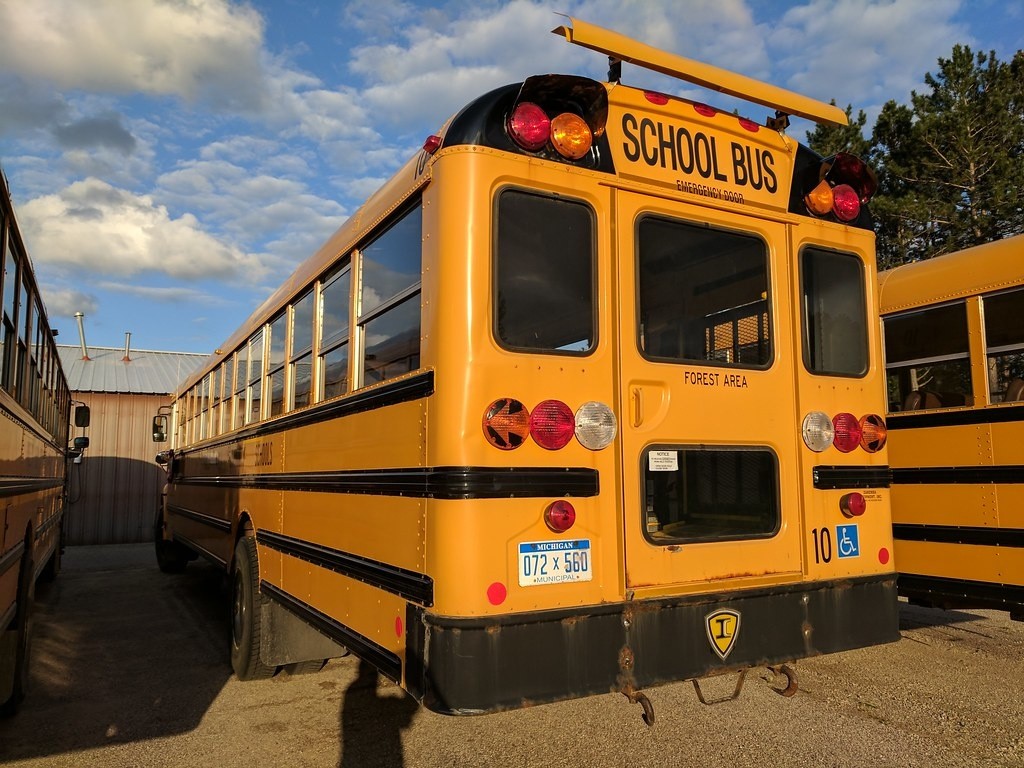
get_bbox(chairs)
[904,390,944,411]
[1001,377,1024,402]
[944,393,970,407]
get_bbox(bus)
[0,172,93,715]
[703,231,1024,618]
[150,19,902,718]
